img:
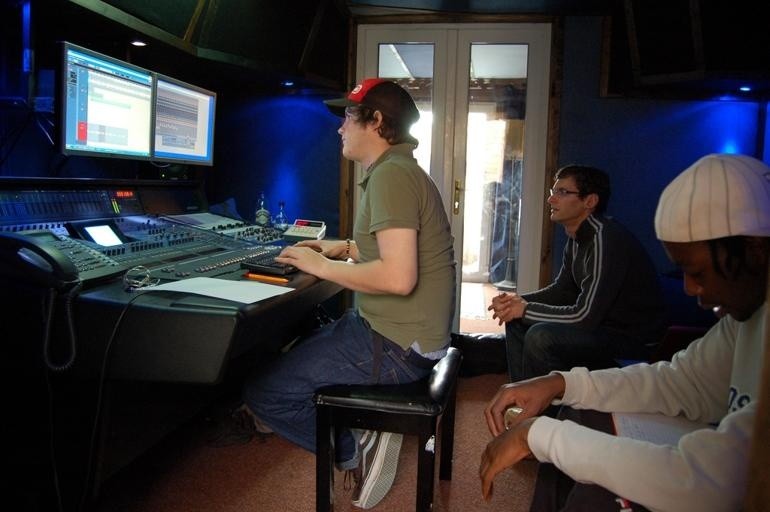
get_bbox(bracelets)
[342,238,351,260]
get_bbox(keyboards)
[241,246,336,275]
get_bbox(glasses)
[549,187,581,196]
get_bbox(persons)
[242,77,457,510]
[476,151,768,512]
[485,162,674,462]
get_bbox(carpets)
[78,370,540,511]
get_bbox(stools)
[312,346,464,512]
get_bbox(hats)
[654,152,770,243]
[324,78,420,128]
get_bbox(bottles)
[275,201,288,229]
[253,191,272,228]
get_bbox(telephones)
[1,230,128,285]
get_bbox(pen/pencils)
[244,273,289,284]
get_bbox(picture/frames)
[623,1,706,89]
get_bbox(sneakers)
[351,429,404,509]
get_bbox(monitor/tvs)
[66,219,131,255]
[62,41,155,162]
[153,72,217,167]
